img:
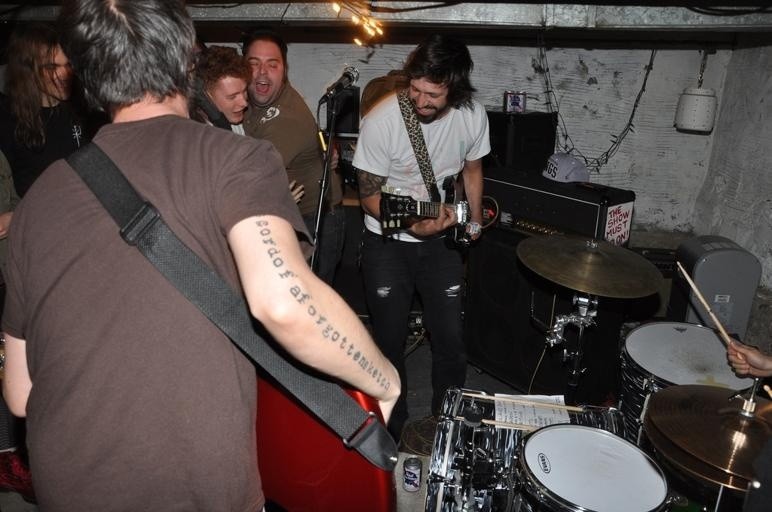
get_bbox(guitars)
[379,174,515,249]
[1,339,398,512]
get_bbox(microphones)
[318,65,360,107]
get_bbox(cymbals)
[517,231,664,298]
[643,385,772,493]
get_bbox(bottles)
[504,89,515,113]
[515,91,526,112]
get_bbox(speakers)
[462,234,618,406]
[482,106,558,174]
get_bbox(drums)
[502,422,688,512]
[618,320,760,445]
[424,387,628,512]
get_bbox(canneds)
[403,455,422,493]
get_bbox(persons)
[0,22,112,268]
[190,46,249,136]
[242,30,345,288]
[0,0,402,512]
[727,341,772,377]
[353,39,492,448]
